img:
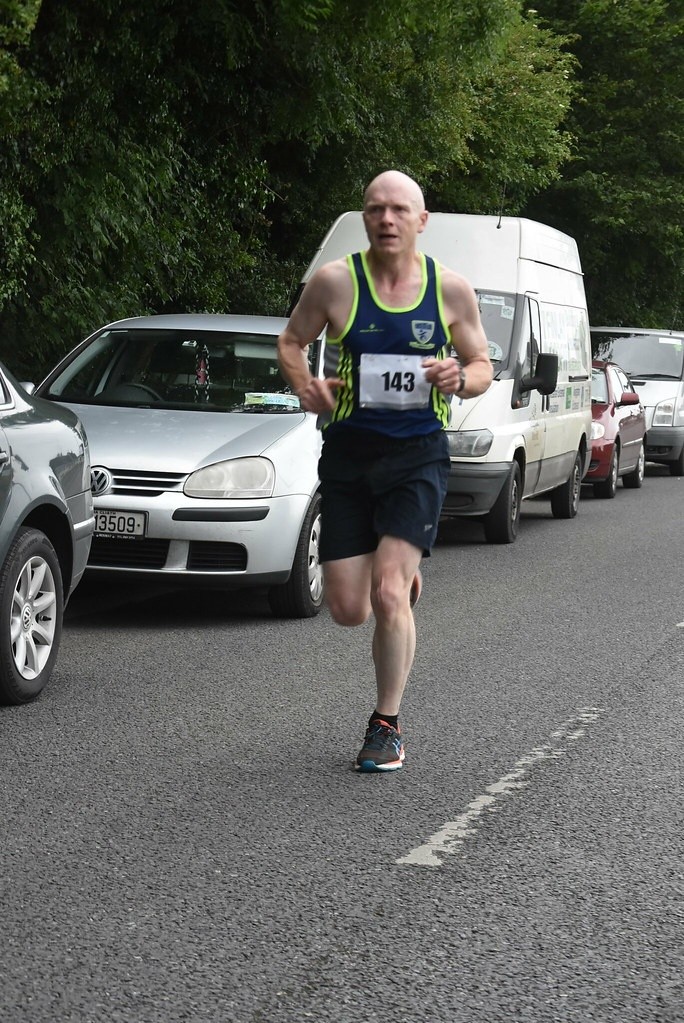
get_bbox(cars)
[580,360,647,499]
[16,313,326,620]
[0,360,95,704]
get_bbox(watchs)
[455,370,466,394]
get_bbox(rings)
[442,380,448,388]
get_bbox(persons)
[275,169,494,772]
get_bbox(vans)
[286,184,594,546]
[589,326,684,476]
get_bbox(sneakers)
[408,567,423,608]
[353,720,406,771]
